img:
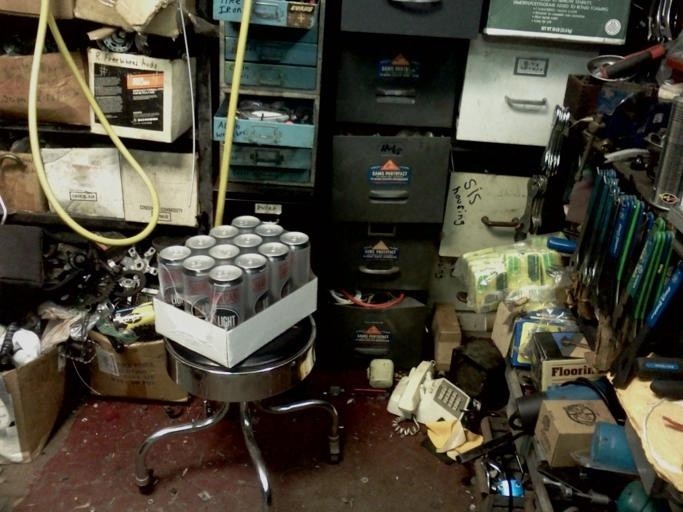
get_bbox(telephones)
[386,360,471,436]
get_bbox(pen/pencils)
[349,387,388,393]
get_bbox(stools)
[133,315,343,512]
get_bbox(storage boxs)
[534,400,618,469]
[490,299,522,356]
[151,274,319,371]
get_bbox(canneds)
[158,215,311,329]
